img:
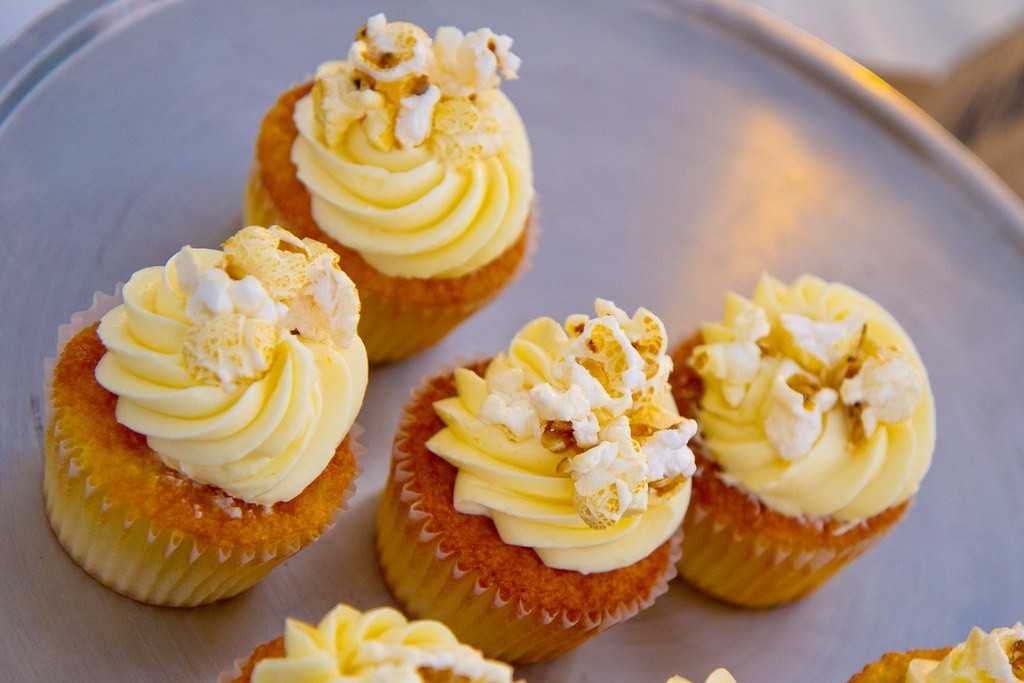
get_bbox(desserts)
[37,9,1024,683]
